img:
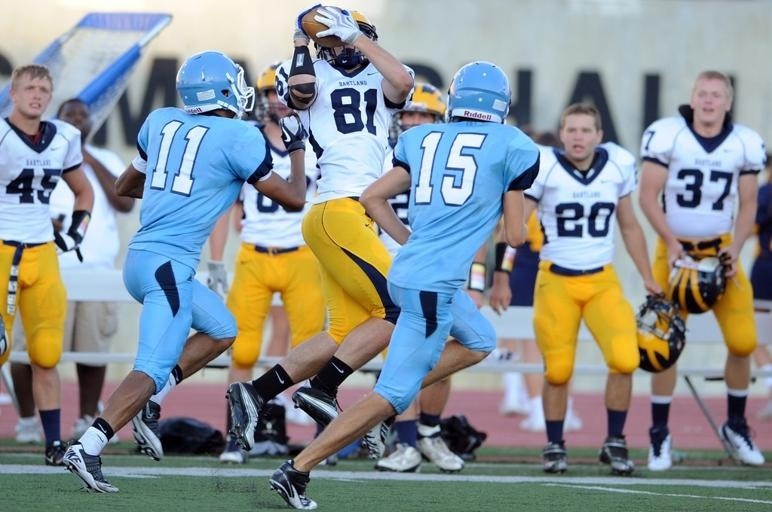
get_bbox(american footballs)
[300,6,350,48]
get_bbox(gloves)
[278,111,307,150]
[52,232,77,257]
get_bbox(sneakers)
[15,417,41,446]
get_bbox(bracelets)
[495,240,516,272]
[69,208,90,244]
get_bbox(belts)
[680,239,721,255]
[3,240,48,314]
[254,245,299,255]
[551,262,603,276]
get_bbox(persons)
[752,153,772,302]
[202,4,564,471]
[267,57,543,511]
[1,65,96,467]
[61,50,309,494]
[11,95,140,451]
[489,102,665,474]
[636,70,767,472]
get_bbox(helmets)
[394,82,445,117]
[670,255,727,314]
[633,298,685,373]
[175,53,255,120]
[313,9,379,66]
[444,61,513,126]
